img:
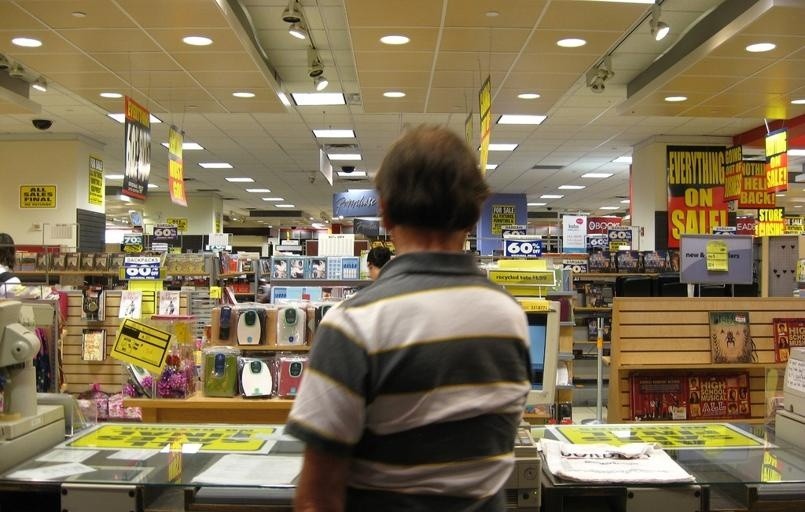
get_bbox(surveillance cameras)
[342,166,355,173]
[32,120,52,131]
[547,207,552,211]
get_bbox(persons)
[778,324,787,336]
[642,392,679,419]
[283,120,532,512]
[779,336,789,348]
[729,389,737,400]
[690,392,699,404]
[166,301,175,314]
[274,260,287,279]
[739,387,747,399]
[740,401,748,412]
[690,378,699,390]
[0,232,22,297]
[292,260,303,277]
[126,301,136,318]
[316,261,325,277]
[728,403,737,415]
[366,245,391,280]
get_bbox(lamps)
[650,5,670,42]
[282,1,329,92]
[0,52,48,93]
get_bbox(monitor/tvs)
[526,300,561,407]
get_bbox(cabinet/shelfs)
[10,251,373,425]
[475,254,576,425]
[754,234,799,298]
[601,296,805,424]
[572,272,661,389]
